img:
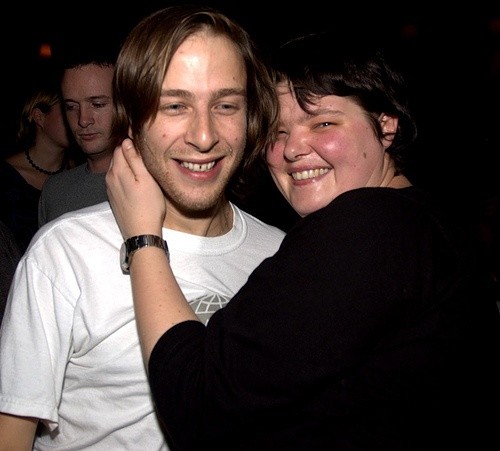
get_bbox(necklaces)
[24,147,67,176]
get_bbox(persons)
[0,82,85,323]
[105,27,500,451]
[38,39,116,227]
[1,7,286,451]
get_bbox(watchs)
[119,234,170,274]
